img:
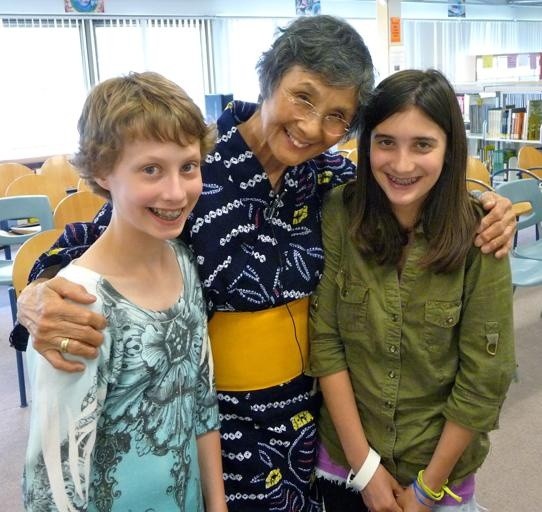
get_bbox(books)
[469,99,542,177]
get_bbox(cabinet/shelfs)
[452,83,542,189]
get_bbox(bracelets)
[346,448,382,491]
[413,470,445,508]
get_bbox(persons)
[302,69,518,512]
[9,13,518,511]
[20,71,228,512]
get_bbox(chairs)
[464,145,542,247]
[333,139,358,164]
[1,153,111,408]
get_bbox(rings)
[58,339,69,355]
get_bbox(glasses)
[280,84,352,134]
